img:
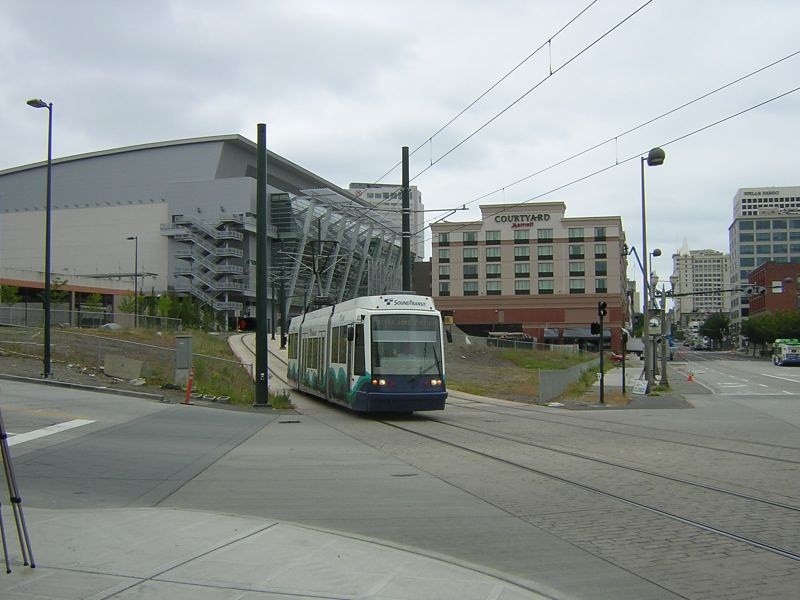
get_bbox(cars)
[596,351,623,363]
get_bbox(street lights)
[648,248,663,375]
[26,97,54,379]
[649,268,679,388]
[125,236,137,329]
[640,147,665,392]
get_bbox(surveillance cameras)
[758,287,767,296]
[744,286,753,296]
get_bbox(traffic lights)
[591,322,601,335]
[758,287,767,295]
[598,302,608,316]
[744,286,754,297]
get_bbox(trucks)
[772,338,800,366]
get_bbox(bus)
[286,240,454,416]
